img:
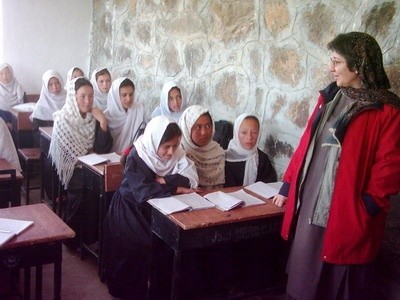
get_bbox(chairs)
[0,169,19,208]
[16,112,40,203]
[23,91,39,102]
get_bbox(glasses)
[330,57,347,64]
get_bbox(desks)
[147,181,298,300]
[0,158,23,184]
[78,154,117,276]
[39,126,51,154]
[1,205,76,299]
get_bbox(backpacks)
[213,120,233,150]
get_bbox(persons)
[0,117,22,173]
[0,64,26,152]
[31,65,279,300]
[272,32,400,300]
